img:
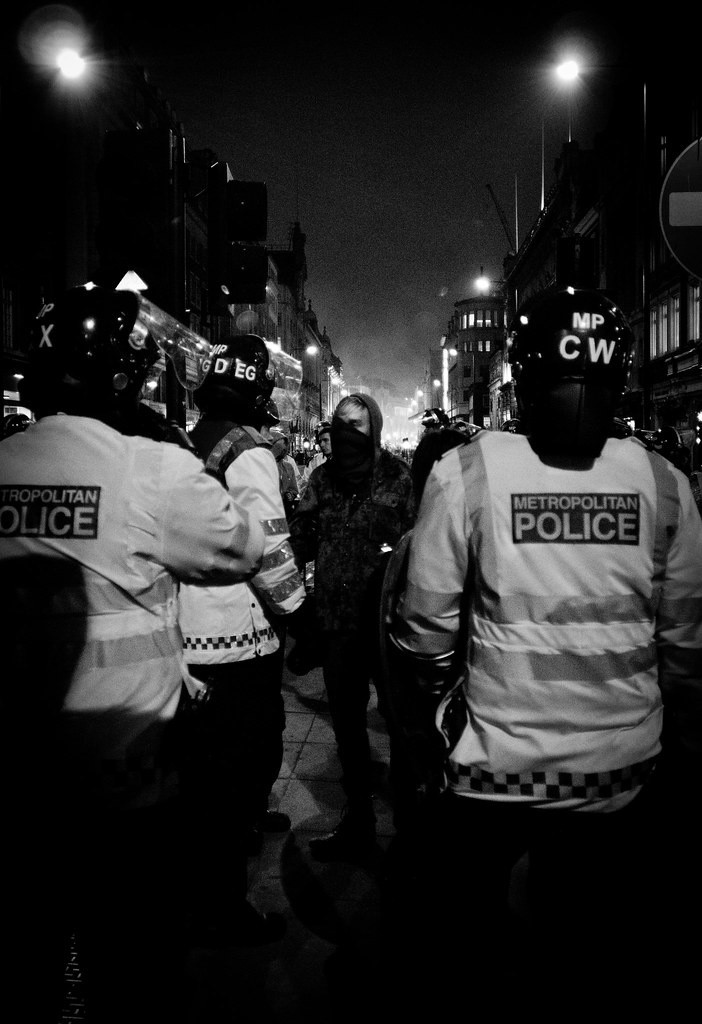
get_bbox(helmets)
[193,334,280,428]
[27,282,162,414]
[609,414,633,438]
[409,408,449,430]
[507,288,635,471]
[648,426,677,447]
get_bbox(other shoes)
[337,730,369,775]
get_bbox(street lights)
[291,346,317,360]
[557,62,579,143]
[318,379,328,421]
[449,348,475,384]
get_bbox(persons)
[1,283,262,1024]
[180,337,702,938]
[390,287,702,814]
